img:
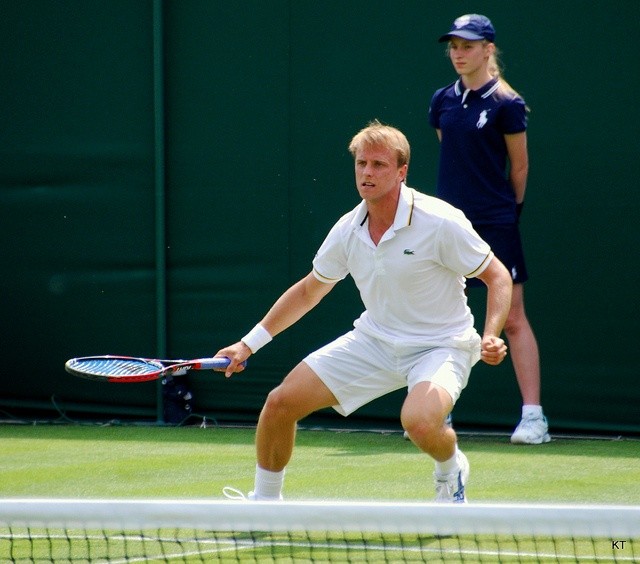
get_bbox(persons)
[209,121,515,540]
[403,14,552,444]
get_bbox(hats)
[438,14,496,43]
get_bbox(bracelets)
[242,324,274,355]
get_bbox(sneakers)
[403,414,453,438]
[435,451,470,505]
[510,412,549,444]
